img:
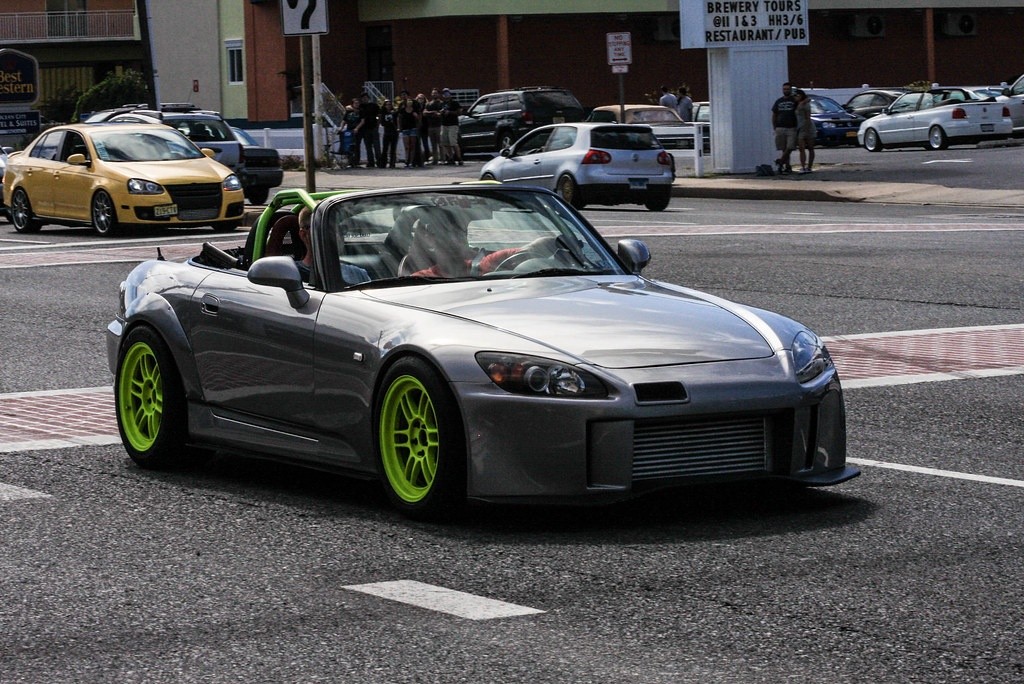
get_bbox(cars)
[232,127,283,204]
[858,73,1024,151]
[587,103,709,149]
[0,143,16,224]
[793,94,864,152]
[688,101,712,150]
[2,123,244,235]
[843,88,914,118]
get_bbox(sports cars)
[105,178,863,518]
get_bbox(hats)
[442,88,450,93]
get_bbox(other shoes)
[775,159,782,174]
[798,167,812,175]
[348,160,464,169]
[783,166,792,175]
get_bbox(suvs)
[480,121,675,211]
[85,102,248,186]
[458,85,586,156]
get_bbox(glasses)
[306,225,348,235]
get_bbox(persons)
[657,85,693,122]
[771,82,816,175]
[616,111,633,124]
[294,206,371,284]
[411,206,557,275]
[337,88,463,171]
